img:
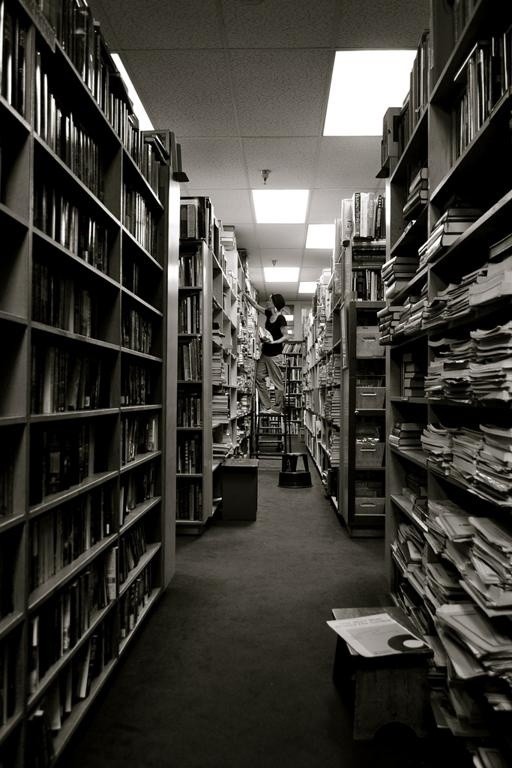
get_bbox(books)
[177,198,257,523]
[372,27,512,768]
[261,340,304,437]
[1,0,169,768]
[307,190,385,514]
[259,327,274,343]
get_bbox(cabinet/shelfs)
[1,0,180,768]
[375,1,512,768]
[179,191,385,542]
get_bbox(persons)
[242,290,290,417]
